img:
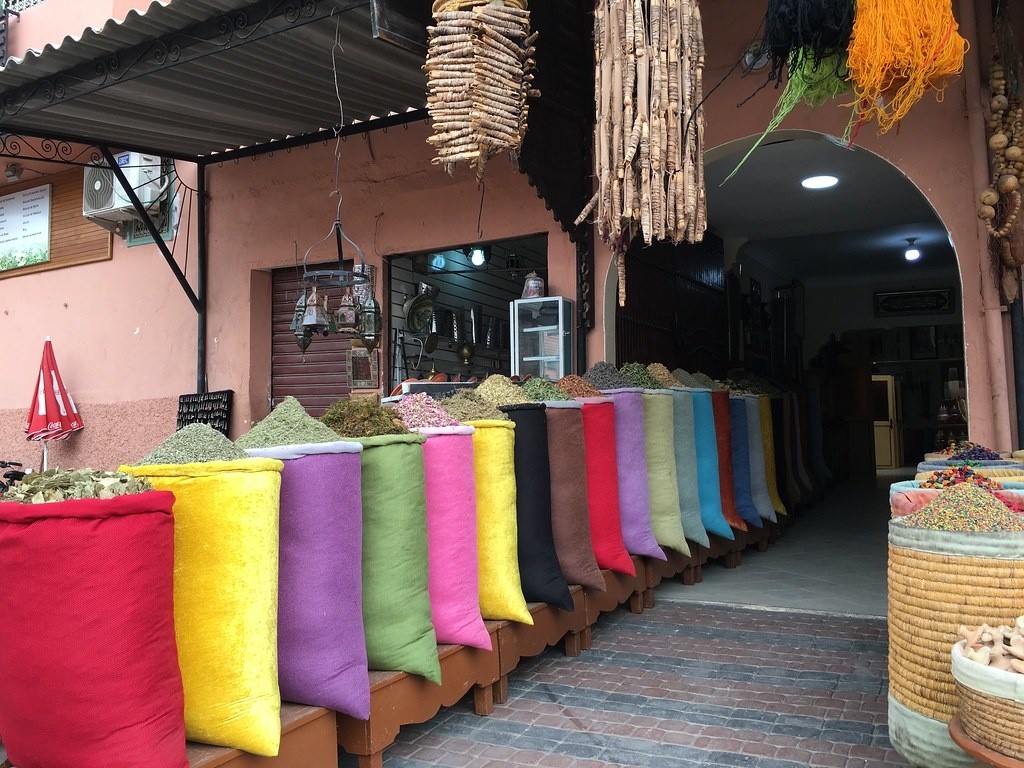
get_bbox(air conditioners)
[82,151,162,221]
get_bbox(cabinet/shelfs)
[508,295,573,381]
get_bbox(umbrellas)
[23,335,84,472]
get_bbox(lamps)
[507,252,522,278]
[4,164,24,183]
[291,279,381,362]
[465,246,492,267]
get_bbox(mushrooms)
[957,615,1024,676]
